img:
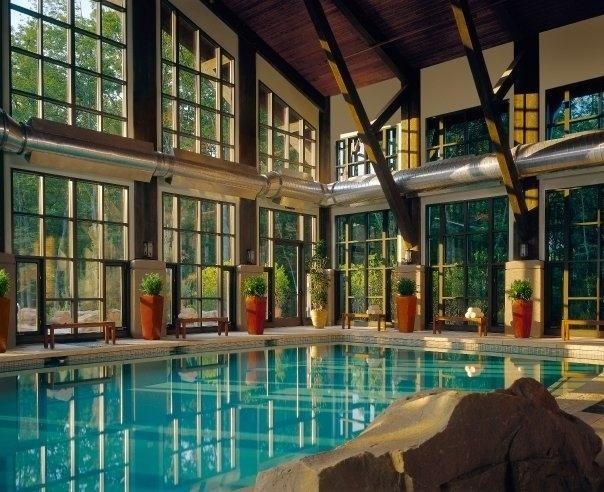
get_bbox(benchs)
[561,319,602,340]
[342,312,385,330]
[175,317,229,339]
[40,321,117,349]
[432,317,486,337]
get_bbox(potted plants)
[310,241,329,328]
[507,280,534,338]
[393,277,416,333]
[138,272,165,340]
[0,270,12,354]
[241,276,268,335]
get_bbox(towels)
[366,305,382,314]
[465,306,484,317]
